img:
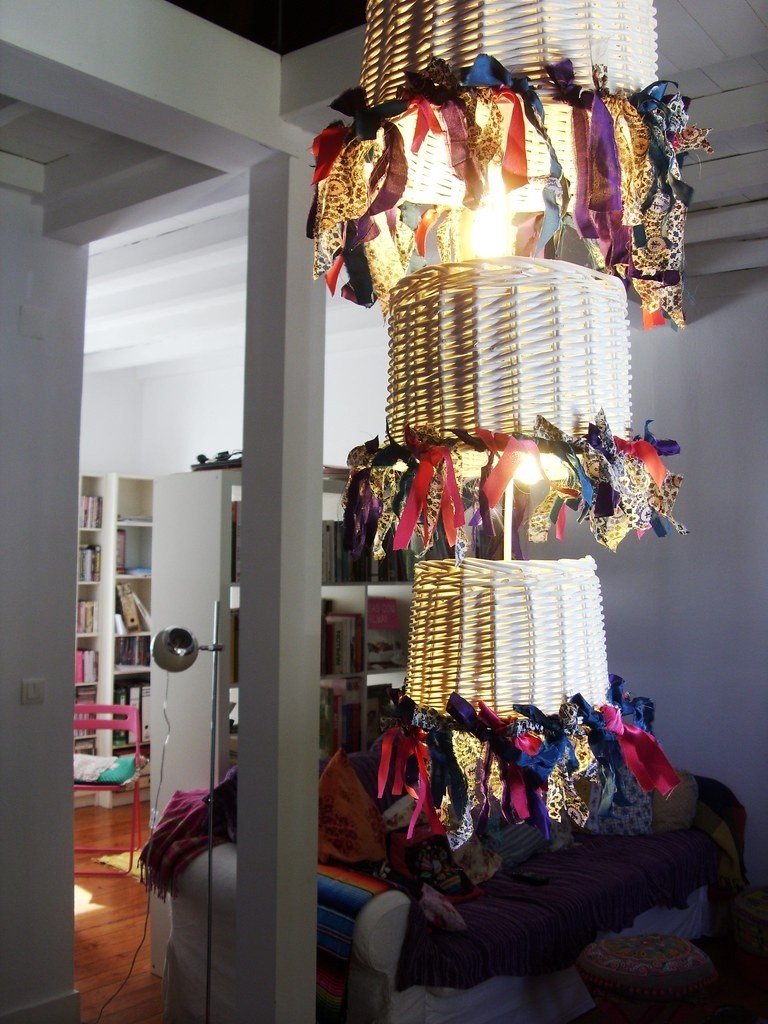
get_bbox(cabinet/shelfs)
[149,471,411,832]
[77,472,155,806]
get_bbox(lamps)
[309,0,712,851]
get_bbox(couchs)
[159,742,747,1024]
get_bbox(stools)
[574,932,718,1024]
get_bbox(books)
[72,494,149,748]
[228,496,408,772]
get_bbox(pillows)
[653,769,699,832]
[574,764,653,836]
[317,746,388,862]
[386,823,489,906]
[474,802,575,867]
